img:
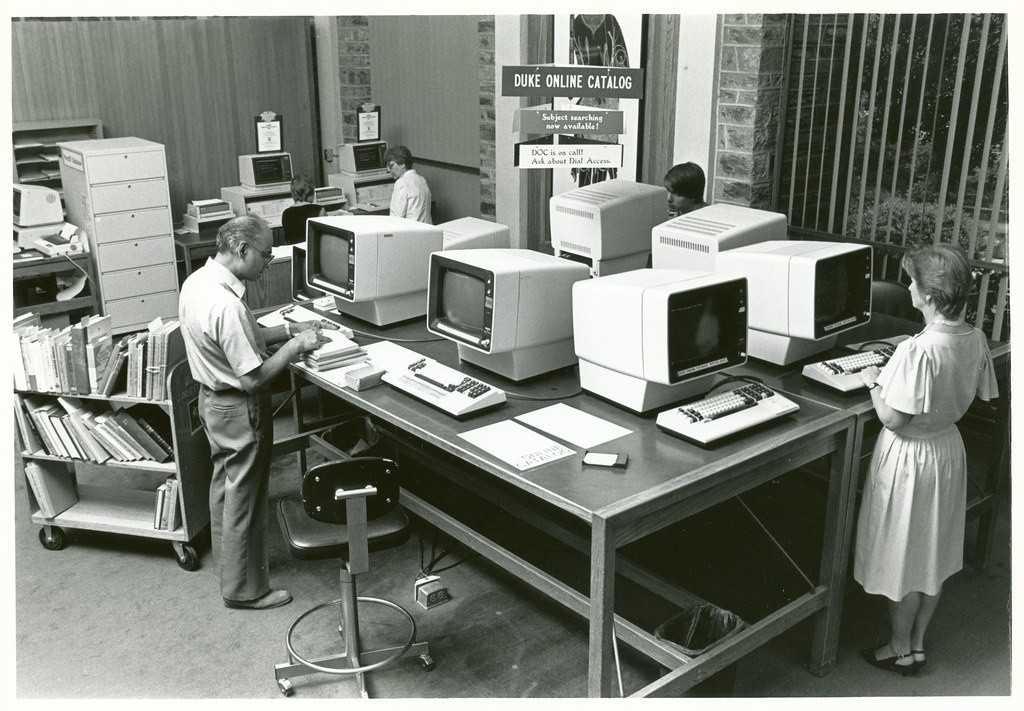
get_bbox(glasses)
[249,242,274,266]
[388,163,395,169]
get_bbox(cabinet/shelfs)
[14,117,313,572]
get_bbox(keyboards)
[657,381,801,450]
[380,356,507,416]
[801,344,898,397]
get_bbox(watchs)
[867,382,878,392]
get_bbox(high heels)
[863,641,927,676]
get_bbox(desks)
[250,300,1011,698]
[172,221,223,276]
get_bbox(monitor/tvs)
[239,141,873,417]
[13,183,64,229]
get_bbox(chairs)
[858,280,921,461]
[274,456,435,699]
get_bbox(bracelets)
[284,324,294,341]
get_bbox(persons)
[854,244,1001,680]
[281,175,329,246]
[570,14,630,182]
[385,146,433,225]
[179,216,332,611]
[665,163,709,216]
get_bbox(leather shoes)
[223,590,291,609]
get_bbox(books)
[304,331,368,372]
[15,154,62,182]
[14,311,186,534]
